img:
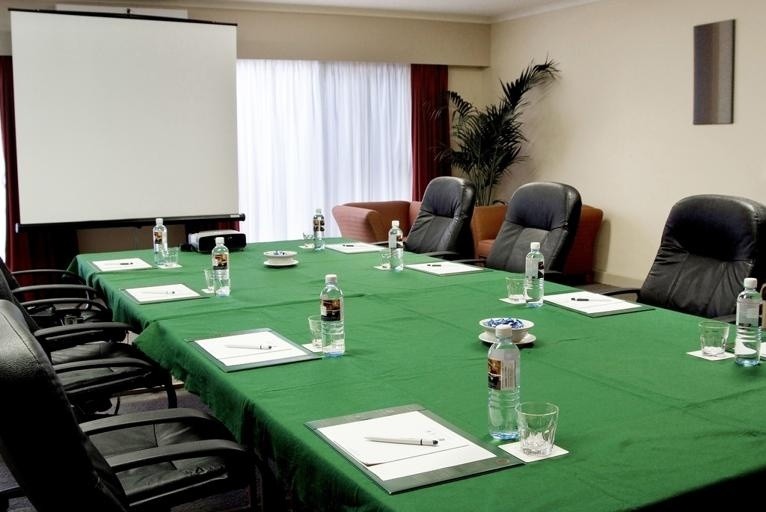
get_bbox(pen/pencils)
[342,243,354,247]
[364,432,438,448]
[119,262,132,265]
[571,298,608,302]
[145,290,175,294]
[427,263,442,267]
[227,344,271,349]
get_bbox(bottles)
[733,277,763,368]
[384,218,404,272]
[484,323,521,440]
[316,272,345,357]
[210,237,232,297]
[151,218,168,265]
[521,240,545,312]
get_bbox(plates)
[262,259,299,267]
[476,330,538,347]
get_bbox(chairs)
[0,298,257,512]
[1,257,180,418]
[470,201,605,284]
[592,193,766,332]
[448,181,584,280]
[330,200,422,243]
[373,175,478,260]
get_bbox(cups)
[161,246,179,271]
[504,273,532,301]
[307,314,323,347]
[698,319,731,356]
[262,249,297,263]
[302,233,315,250]
[514,399,560,457]
[477,315,535,340]
[311,210,328,248]
[202,265,218,291]
[377,249,393,269]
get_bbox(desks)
[62,238,764,512]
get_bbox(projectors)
[187,229,246,253]
